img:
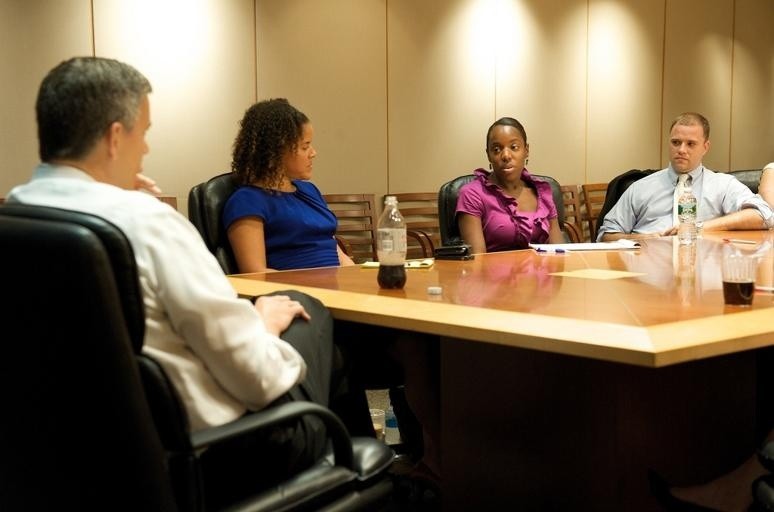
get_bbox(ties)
[673,173,693,227]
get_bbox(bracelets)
[696,219,705,234]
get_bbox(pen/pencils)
[536,248,566,253]
[722,238,758,244]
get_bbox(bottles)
[385,402,400,444]
[676,244,696,305]
[678,188,697,244]
[376,196,407,289]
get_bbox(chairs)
[1,202,412,512]
[187,172,245,274]
[595,169,662,242]
[439,175,564,247]
[726,169,763,194]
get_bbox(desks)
[226,229,773,367]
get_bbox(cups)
[720,255,757,307]
[369,409,385,441]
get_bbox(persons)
[454,117,563,255]
[671,427,774,512]
[11,57,348,489]
[596,111,771,243]
[454,254,564,311]
[223,98,419,464]
[624,241,769,298]
